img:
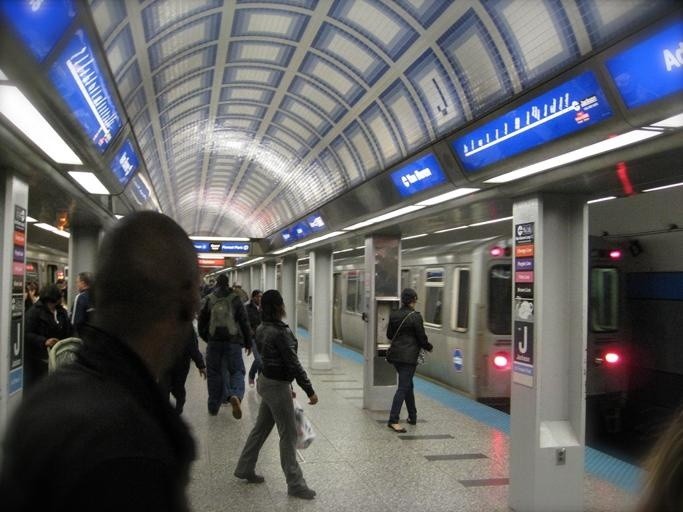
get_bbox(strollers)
[42,334,86,377]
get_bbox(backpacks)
[206,292,239,342]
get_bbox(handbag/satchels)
[385,347,393,364]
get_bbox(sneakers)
[229,395,242,419]
[234,470,264,483]
[288,488,316,499]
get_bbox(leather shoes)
[406,418,416,425]
[387,424,407,433]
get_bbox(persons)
[26,281,40,305]
[159,320,208,417]
[199,278,215,296]
[53,270,67,306]
[198,275,253,420]
[1,210,201,511]
[230,283,250,302]
[244,290,261,388]
[232,289,317,499]
[24,287,32,311]
[18,282,70,387]
[386,288,433,432]
[68,271,93,338]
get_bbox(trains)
[25,243,71,304]
[275,236,631,406]
